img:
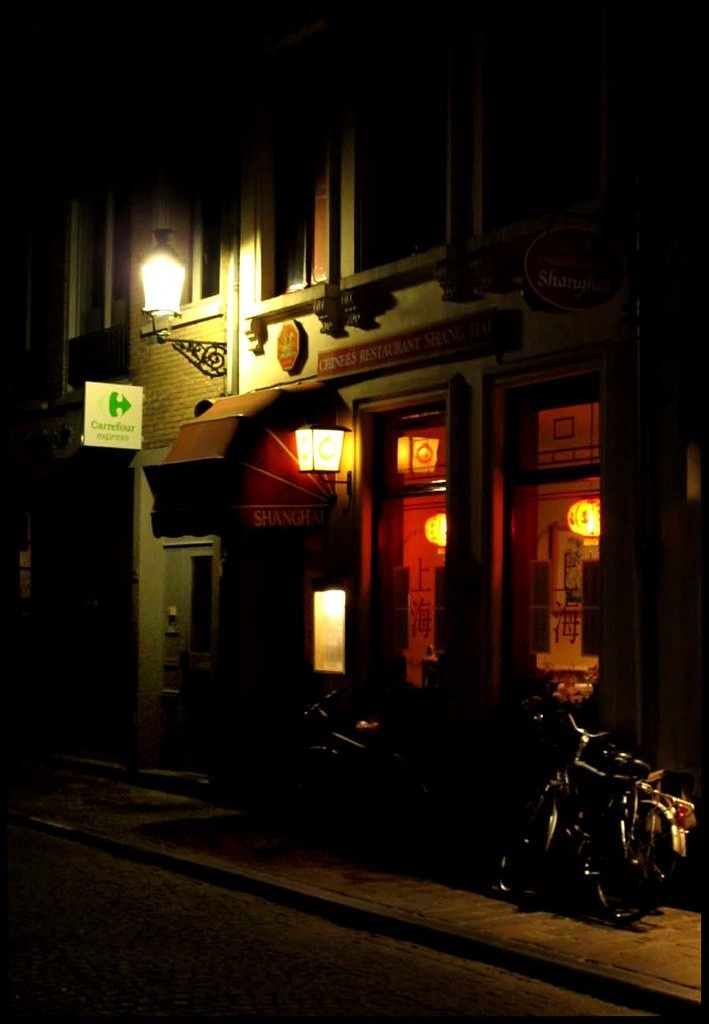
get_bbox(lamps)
[138,228,226,378]
[289,414,355,498]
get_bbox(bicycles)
[499,708,695,924]
[300,686,408,771]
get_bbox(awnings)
[140,383,352,538]
